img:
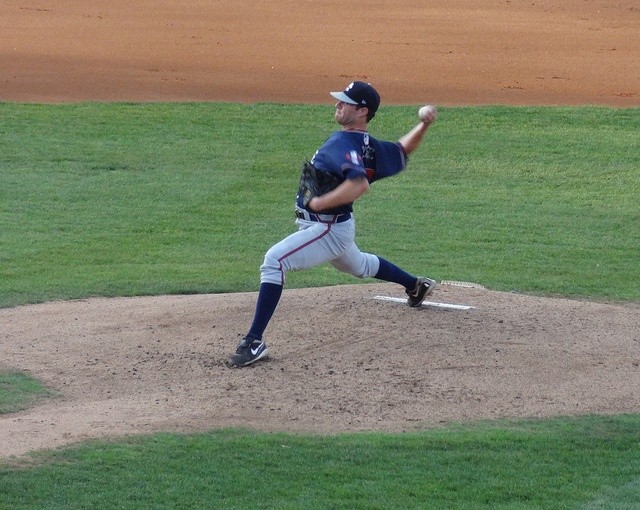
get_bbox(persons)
[226,80,438,368]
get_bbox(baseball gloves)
[300,160,339,213]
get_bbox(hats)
[329,81,380,112]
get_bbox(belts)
[294,209,351,224]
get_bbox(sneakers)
[231,336,268,366]
[406,276,436,306]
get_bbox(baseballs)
[419,106,433,119]
